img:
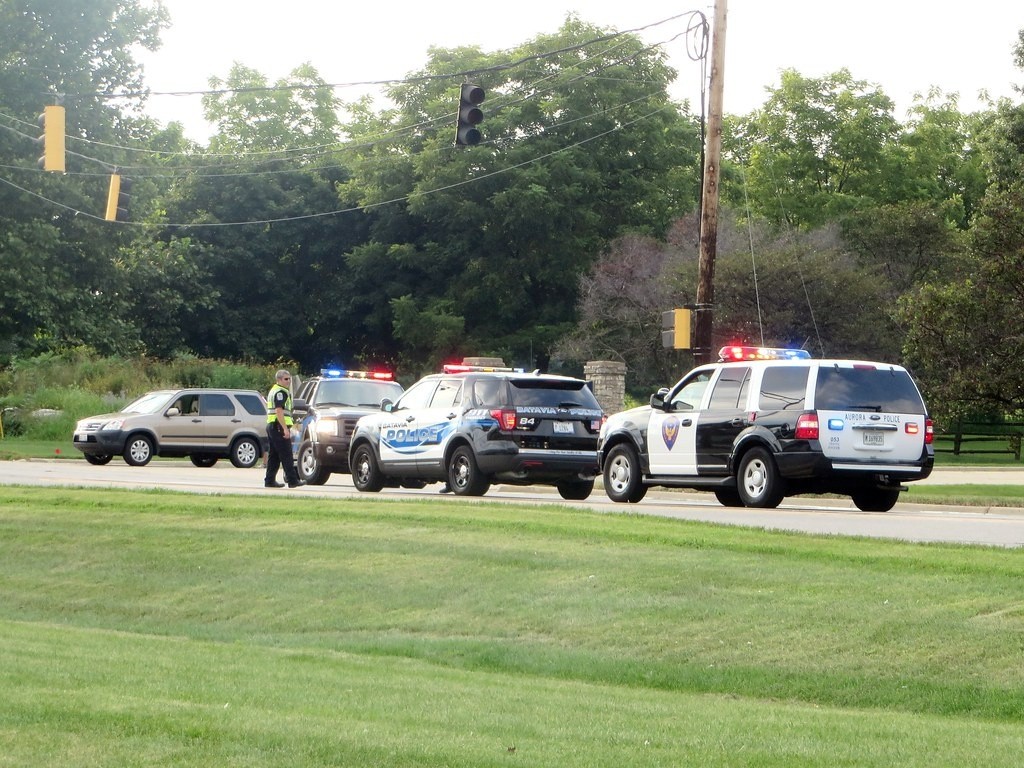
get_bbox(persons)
[265,370,307,488]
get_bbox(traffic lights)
[661,308,692,350]
[453,83,485,148]
[35,106,66,174]
[105,174,134,222]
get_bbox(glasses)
[281,377,290,380]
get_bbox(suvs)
[347,365,609,500]
[596,346,936,511]
[289,368,406,485]
[72,387,271,468]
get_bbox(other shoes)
[265,481,285,487]
[288,480,306,487]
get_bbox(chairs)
[174,400,182,416]
[190,400,198,416]
